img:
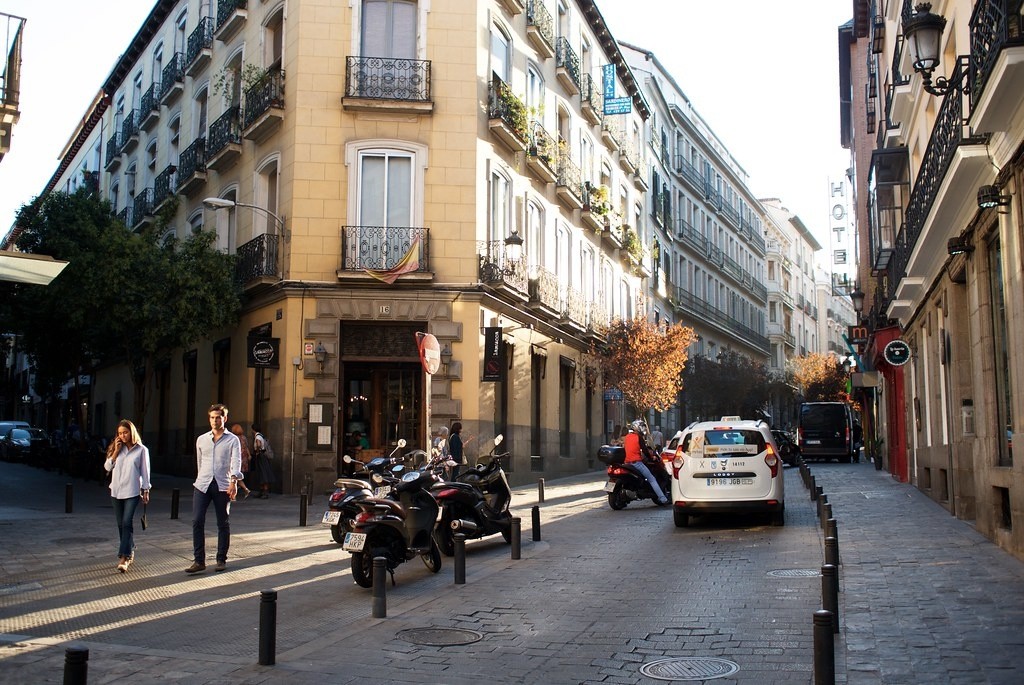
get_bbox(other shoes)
[258,491,268,497]
[214,559,226,573]
[117,562,128,573]
[244,488,251,500]
[663,500,672,508]
[184,562,206,572]
[128,557,133,564]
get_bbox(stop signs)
[419,333,443,376]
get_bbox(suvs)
[670,419,789,528]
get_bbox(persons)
[346,430,369,475]
[852,420,863,463]
[183,404,243,572]
[610,423,628,447]
[449,423,475,482]
[231,423,252,501]
[434,426,450,473]
[103,419,152,571]
[250,424,273,499]
[624,420,669,504]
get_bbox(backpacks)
[257,433,275,460]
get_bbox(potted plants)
[866,436,885,470]
[208,63,280,107]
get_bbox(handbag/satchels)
[462,452,468,465]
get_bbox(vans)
[796,401,858,463]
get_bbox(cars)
[0,420,31,443]
[660,430,681,476]
[0,428,52,464]
[723,429,802,467]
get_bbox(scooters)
[322,433,514,588]
[597,443,672,510]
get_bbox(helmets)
[630,420,648,436]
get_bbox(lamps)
[203,198,286,237]
[440,344,453,378]
[478,229,524,282]
[580,349,590,353]
[849,287,868,324]
[948,236,978,255]
[525,323,539,329]
[553,337,565,343]
[314,340,328,373]
[900,1,970,96]
[843,354,865,373]
[977,185,1012,209]
